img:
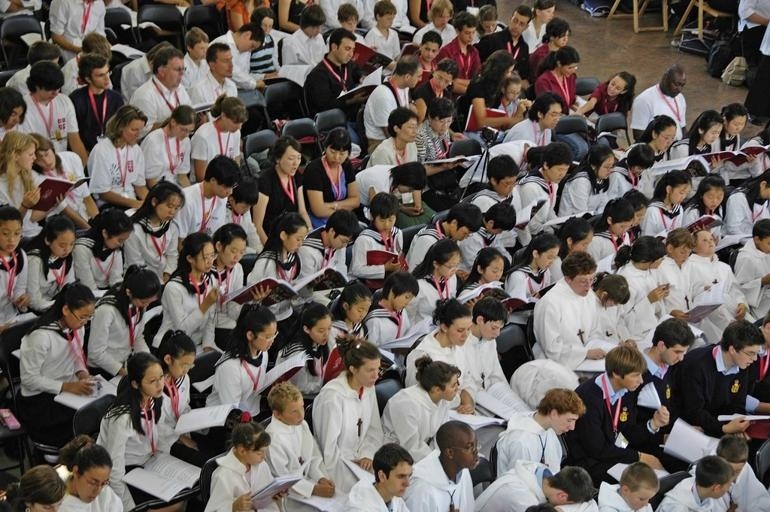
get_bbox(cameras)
[480,126,499,145]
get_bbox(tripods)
[459,143,490,202]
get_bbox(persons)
[0,0,768,486]
[596,461,660,512]
[200,410,291,512]
[309,339,386,494]
[91,352,201,511]
[55,433,125,511]
[652,453,735,512]
[402,419,483,511]
[0,465,67,512]
[262,376,347,512]
[687,433,770,512]
[344,442,416,512]
[473,459,598,512]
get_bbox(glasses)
[69,310,95,325]
[452,444,482,451]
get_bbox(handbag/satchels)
[706,38,748,86]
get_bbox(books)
[292,479,343,510]
[242,474,298,505]
[123,450,204,503]
